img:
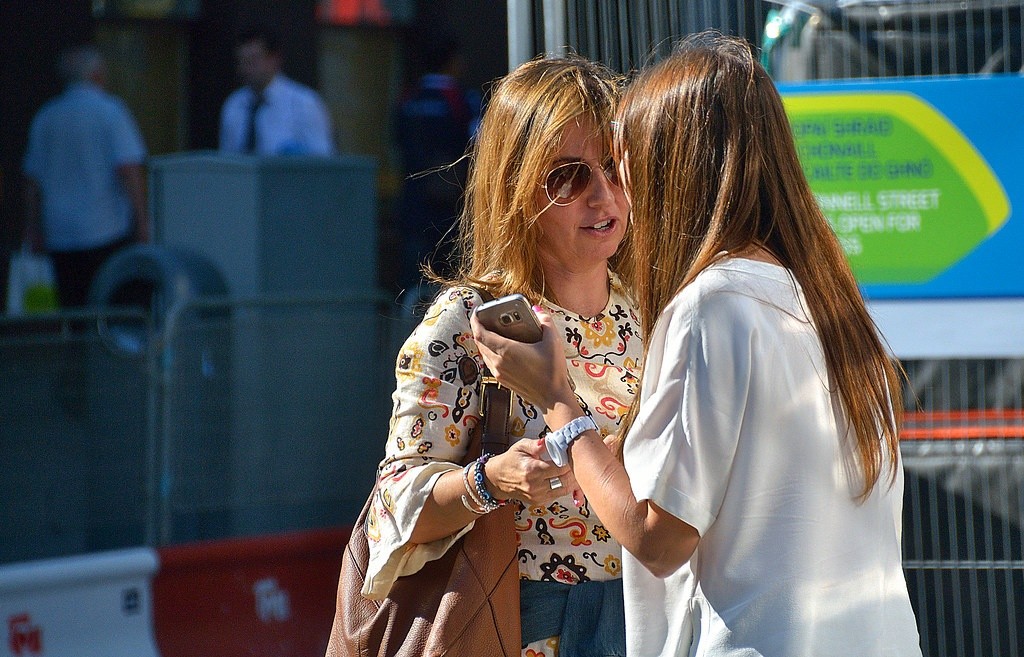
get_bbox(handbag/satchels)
[324,284,521,657]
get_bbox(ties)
[242,93,265,157]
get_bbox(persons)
[16,46,149,413]
[215,27,334,156]
[468,47,924,657]
[359,56,645,657]
[391,49,483,325]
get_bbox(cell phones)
[476,294,576,391]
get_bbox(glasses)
[535,154,622,206]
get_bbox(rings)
[549,476,563,489]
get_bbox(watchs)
[544,415,599,467]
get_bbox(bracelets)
[461,453,512,516]
